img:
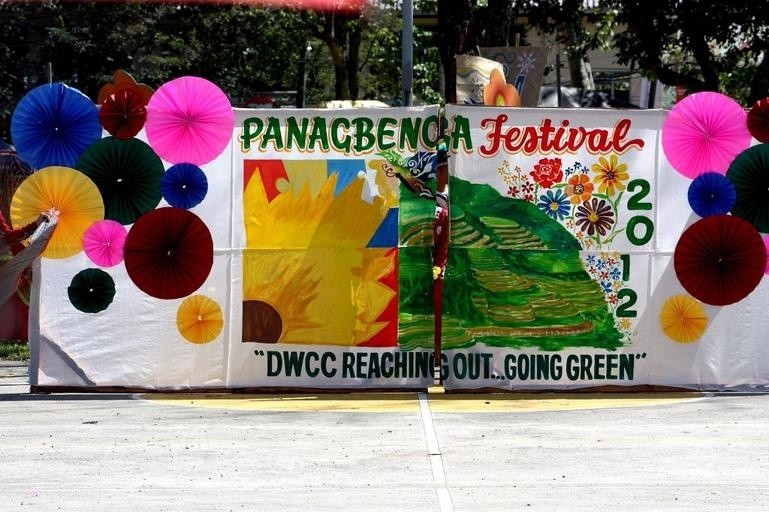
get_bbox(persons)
[0,211,52,253]
